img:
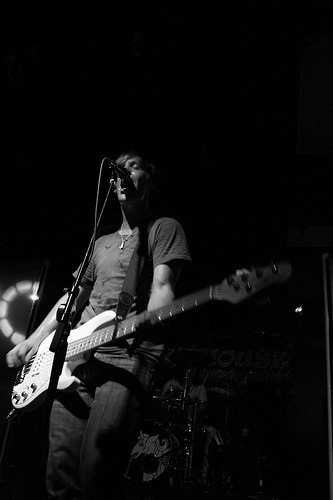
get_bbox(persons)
[5,152,192,500]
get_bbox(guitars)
[10,258,293,414]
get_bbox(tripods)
[197,365,252,500]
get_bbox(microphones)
[105,157,130,180]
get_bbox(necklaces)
[118,225,138,250]
